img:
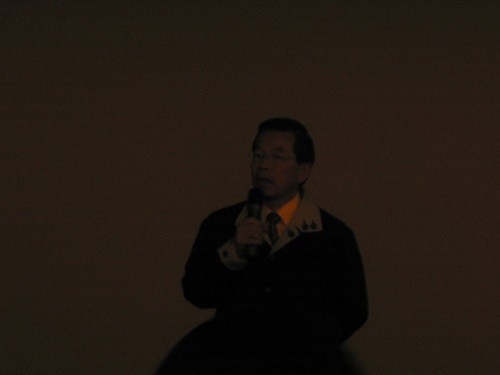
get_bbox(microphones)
[248,187,263,257]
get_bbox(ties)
[266,212,280,244]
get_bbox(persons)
[181,117,369,374]
